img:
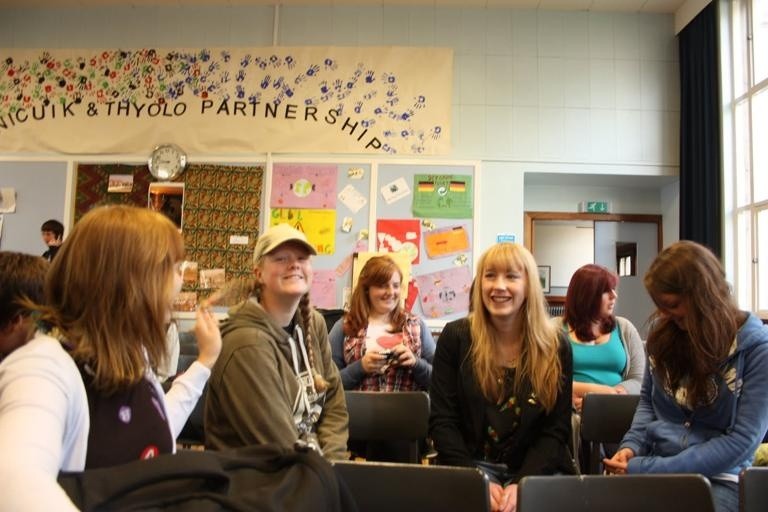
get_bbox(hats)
[253,225,317,262]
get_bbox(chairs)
[159,331,768,512]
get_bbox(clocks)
[147,140,191,181]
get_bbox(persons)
[1,203,223,512]
[1,251,50,361]
[428,242,574,512]
[549,265,646,474]
[204,224,368,463]
[41,220,63,262]
[328,258,437,393]
[601,240,768,512]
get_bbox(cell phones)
[378,350,392,360]
